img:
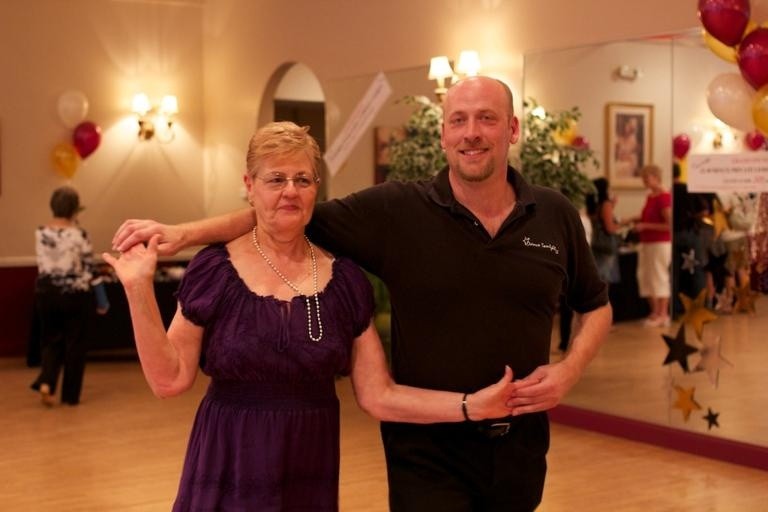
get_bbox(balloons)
[698,0,768,152]
[570,135,592,151]
[673,133,691,161]
[56,90,90,131]
[673,135,691,158]
[552,119,578,150]
[49,143,83,179]
[70,121,102,158]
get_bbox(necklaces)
[251,223,324,343]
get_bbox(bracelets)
[461,392,471,424]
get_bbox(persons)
[100,119,541,512]
[584,176,637,287]
[30,185,111,408]
[614,115,642,178]
[673,164,768,319]
[109,75,615,512]
[622,164,674,329]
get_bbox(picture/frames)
[604,103,653,191]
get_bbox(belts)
[433,411,545,438]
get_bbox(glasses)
[253,172,320,188]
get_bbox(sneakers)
[641,313,671,328]
[39,383,59,409]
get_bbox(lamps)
[428,51,480,101]
[129,91,179,143]
